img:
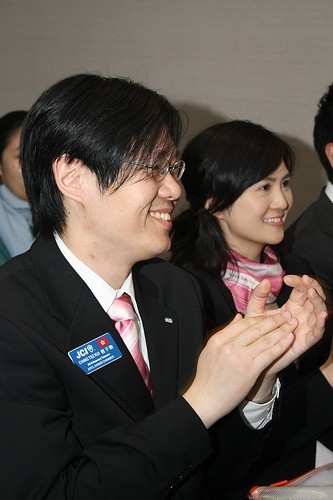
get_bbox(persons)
[0,108,44,267]
[0,72,327,500]
[277,85,332,451]
[163,120,332,500]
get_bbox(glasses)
[123,160,185,182]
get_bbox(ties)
[107,292,152,394]
